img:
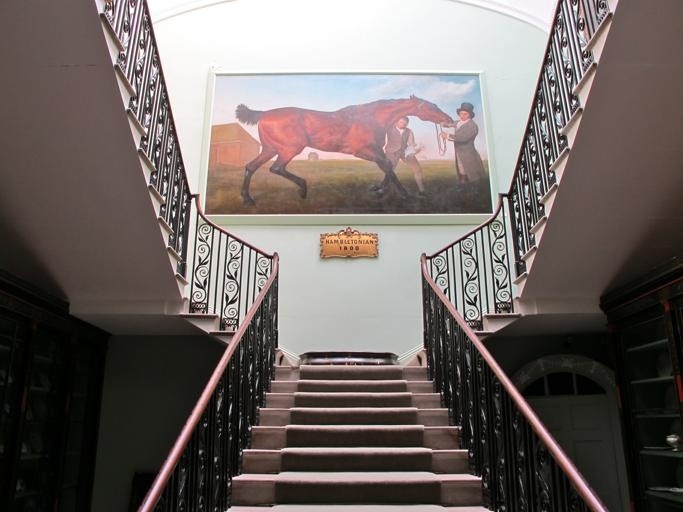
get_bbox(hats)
[457,103,475,118]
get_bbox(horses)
[235,93,455,208]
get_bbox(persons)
[381,116,425,195]
[442,102,485,187]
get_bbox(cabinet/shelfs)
[599,254,683,512]
[0,267,115,512]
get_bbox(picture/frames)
[197,67,499,226]
